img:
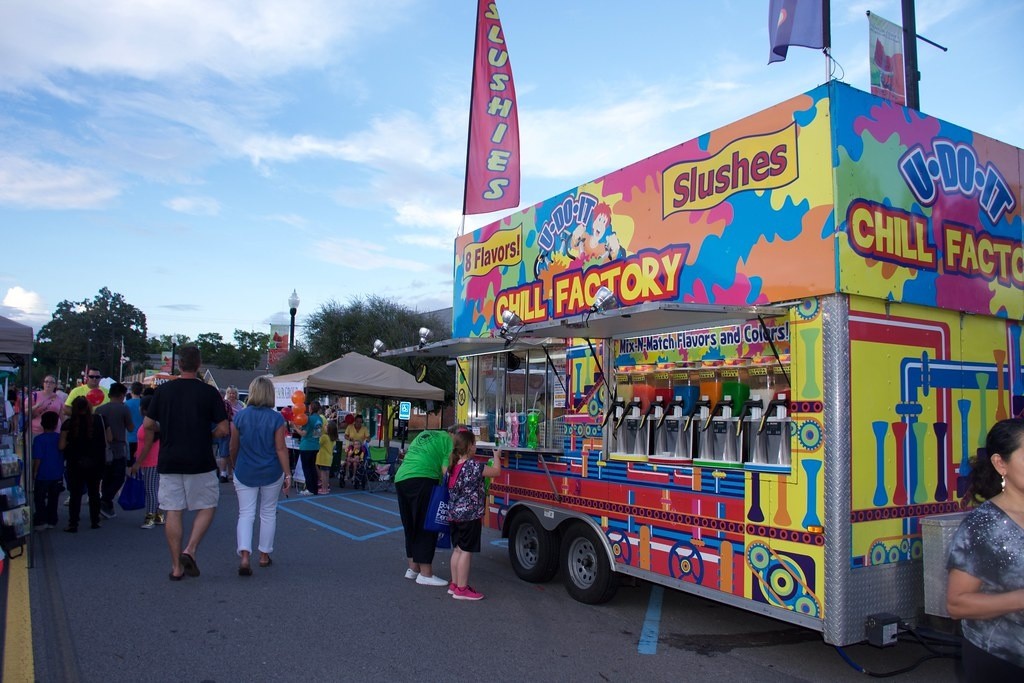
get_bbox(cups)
[497,432,508,447]
[493,434,498,446]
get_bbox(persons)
[4,344,408,579]
[394,424,503,600]
[947,416,1024,683]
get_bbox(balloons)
[293,402,306,415]
[345,414,355,424]
[281,407,293,420]
[292,413,308,427]
[291,390,306,403]
[86,388,105,406]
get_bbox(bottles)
[471,414,489,444]
[510,412,520,448]
[527,411,540,449]
[518,412,527,448]
[505,412,512,448]
[484,457,494,495]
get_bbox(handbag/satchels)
[99,414,113,462]
[292,454,305,483]
[436,531,451,549]
[423,470,451,533]
[118,468,145,511]
[19,391,37,433]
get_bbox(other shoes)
[63,496,71,505]
[91,523,103,529]
[48,524,55,528]
[140,513,154,528]
[34,524,47,530]
[64,527,78,533]
[100,509,116,519]
[154,514,165,524]
[300,480,331,495]
[219,475,229,482]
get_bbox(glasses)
[88,375,101,379]
[44,380,56,384]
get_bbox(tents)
[0,314,34,568]
[0,352,19,401]
[268,351,445,493]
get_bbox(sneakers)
[405,568,418,579]
[416,573,448,586]
[452,585,484,600]
[447,583,475,594]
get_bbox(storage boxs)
[284,437,298,447]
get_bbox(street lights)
[287,288,299,373]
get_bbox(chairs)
[328,441,343,478]
[365,447,401,493]
[287,447,300,490]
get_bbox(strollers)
[339,438,371,491]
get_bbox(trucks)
[377,85,1021,648]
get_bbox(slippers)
[239,567,252,575]
[169,572,184,581]
[260,555,272,567]
[179,553,200,577]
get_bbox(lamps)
[419,327,437,345]
[506,351,520,371]
[500,310,523,335]
[369,339,388,358]
[591,284,618,315]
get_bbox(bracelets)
[285,474,293,479]
